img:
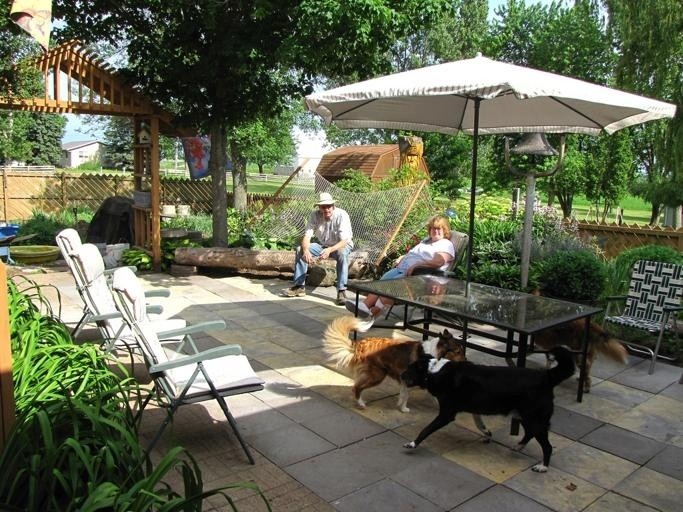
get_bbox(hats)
[313,193,335,207]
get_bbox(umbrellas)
[303,51,677,297]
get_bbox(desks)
[344,273,603,437]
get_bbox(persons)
[345,215,455,320]
[281,193,353,305]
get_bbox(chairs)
[112,266,264,465]
[55,228,137,340]
[384,230,469,325]
[595,260,682,375]
[69,243,187,376]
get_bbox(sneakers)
[280,286,305,296]
[336,291,346,305]
[345,300,372,318]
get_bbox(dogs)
[320,310,467,413]
[530,288,628,393]
[401,343,575,472]
